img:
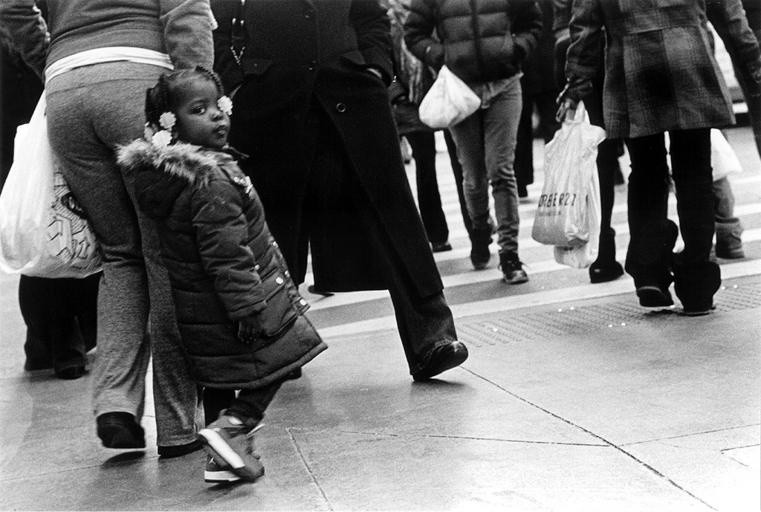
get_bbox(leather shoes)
[413,340,468,382]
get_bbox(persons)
[115,65,328,483]
[385,1,760,313]
[216,1,468,384]
[1,1,217,458]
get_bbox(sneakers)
[470,231,489,268]
[97,412,145,449]
[158,412,264,484]
[636,276,713,314]
[500,252,527,283]
[715,243,744,259]
